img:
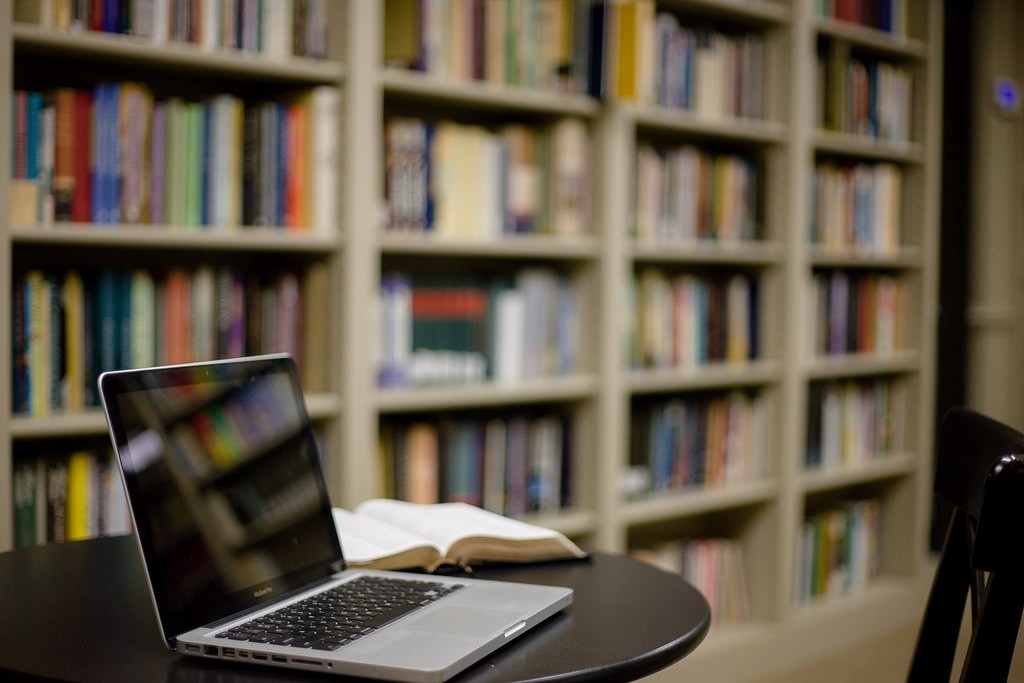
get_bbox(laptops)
[98,351,575,683]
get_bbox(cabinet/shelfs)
[0,0,943,683]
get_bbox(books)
[117,364,322,593]
[816,0,893,32]
[15,0,329,58]
[631,537,748,633]
[816,41,914,142]
[637,145,749,238]
[11,82,341,235]
[634,392,768,493]
[655,13,765,120]
[380,413,570,517]
[385,0,608,104]
[385,116,594,234]
[631,267,757,367]
[807,377,905,468]
[817,272,902,354]
[378,266,575,389]
[13,263,326,418]
[800,499,884,605]
[12,449,132,543]
[813,161,900,247]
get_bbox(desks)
[1,533,711,683]
